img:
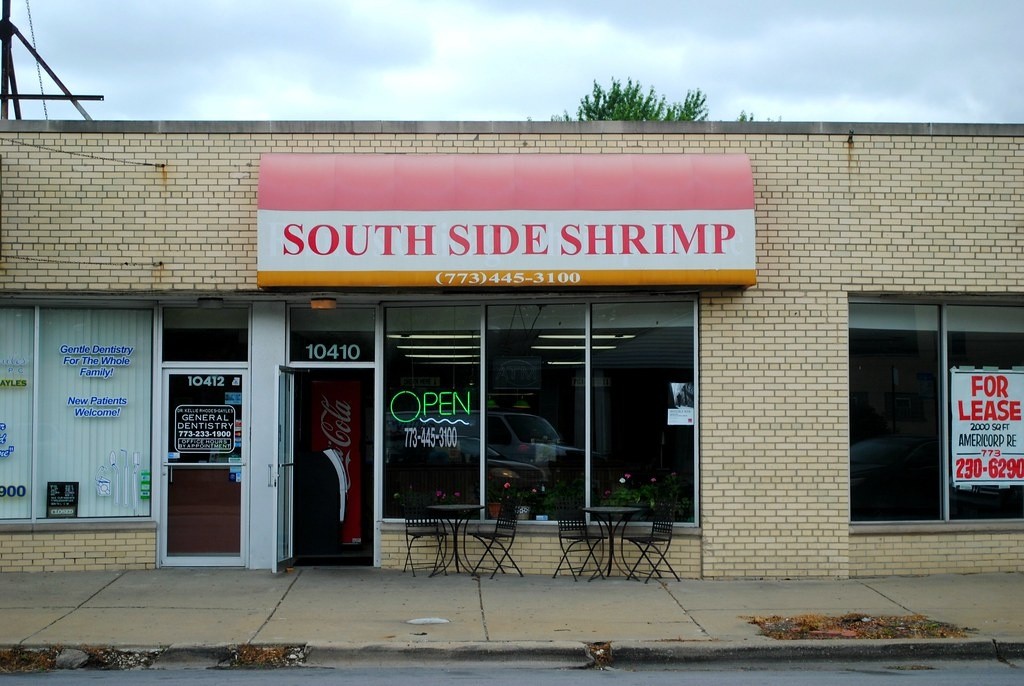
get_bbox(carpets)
[293,557,373,566]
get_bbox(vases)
[515,505,531,520]
[487,503,506,520]
[628,503,650,521]
[548,501,564,520]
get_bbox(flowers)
[395,472,689,521]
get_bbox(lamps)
[311,297,336,308]
[197,298,223,309]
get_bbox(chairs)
[403,496,523,579]
[552,500,680,584]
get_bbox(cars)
[384,408,612,522]
[850,433,941,521]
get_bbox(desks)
[581,507,642,582]
[426,504,486,578]
